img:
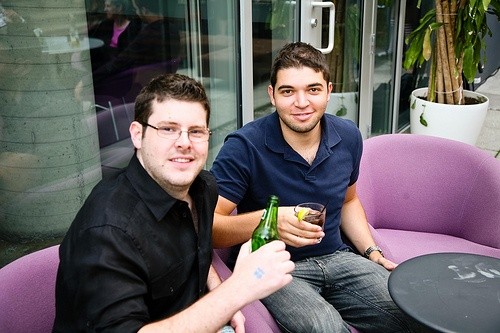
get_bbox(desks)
[387,253,500,333]
[38,37,104,75]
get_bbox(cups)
[293,202,326,243]
[70,29,79,49]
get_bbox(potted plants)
[404,0,493,147]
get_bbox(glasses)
[143,121,213,142]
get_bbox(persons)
[88,0,183,98]
[207,40,434,333]
[50,72,296,333]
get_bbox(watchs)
[363,245,385,259]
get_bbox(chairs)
[1,132,500,333]
[96,56,180,149]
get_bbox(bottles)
[251,195,280,252]
